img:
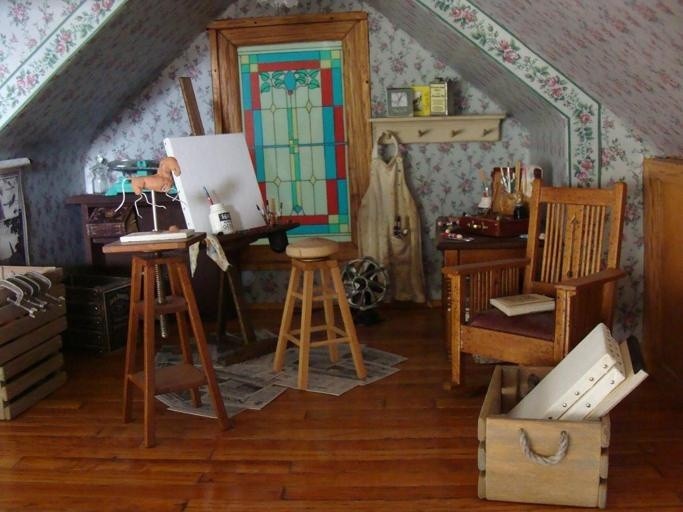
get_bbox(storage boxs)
[475,360,612,506]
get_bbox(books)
[408,84,430,117]
[487,293,557,317]
[385,87,414,119]
[118,228,194,243]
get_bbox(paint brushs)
[203,187,214,205]
[256,200,283,225]
[500,161,511,194]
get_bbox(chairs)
[445,179,633,390]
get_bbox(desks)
[433,216,555,317]
[232,242,355,314]
[64,191,190,267]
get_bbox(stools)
[121,245,231,448]
[268,241,369,392]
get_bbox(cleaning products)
[429,74,454,117]
